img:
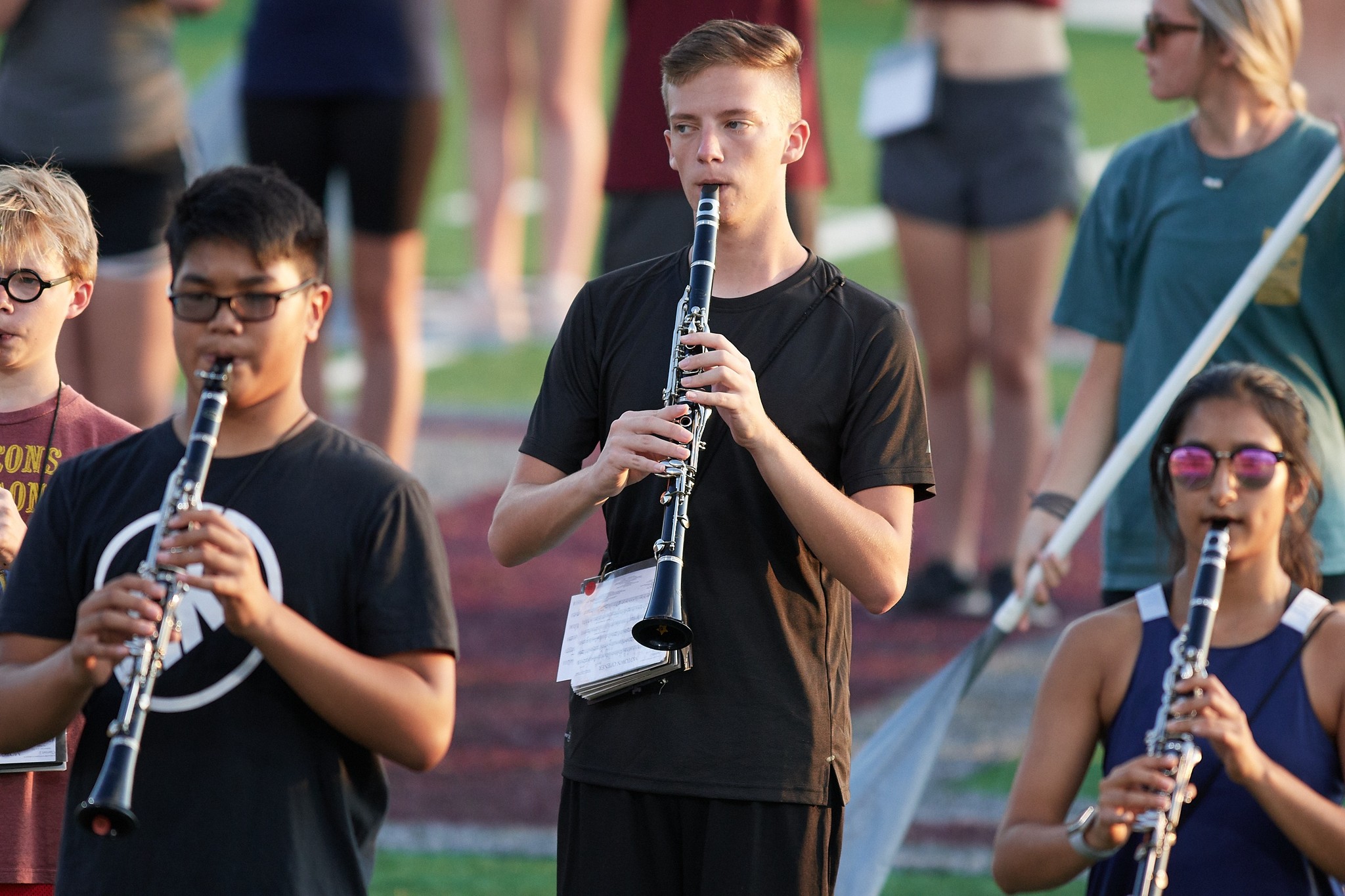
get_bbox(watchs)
[1065,806,1121,861]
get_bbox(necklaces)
[1196,121,1271,189]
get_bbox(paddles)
[833,135,1345,896]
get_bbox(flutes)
[69,354,235,850]
[1128,521,1231,896]
[631,179,723,651]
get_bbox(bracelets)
[1019,487,1078,521]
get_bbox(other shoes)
[990,564,1060,626]
[906,561,992,621]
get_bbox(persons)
[1,165,461,896]
[595,1,830,283]
[457,1,610,346]
[993,362,1345,896]
[1014,0,1343,606]
[0,2,217,426]
[237,0,449,483]
[488,20,941,895]
[873,1,1082,629]
[0,165,144,896]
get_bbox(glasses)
[1162,441,1295,490]
[168,277,319,324]
[0,269,78,303]
[1144,16,1201,52]
